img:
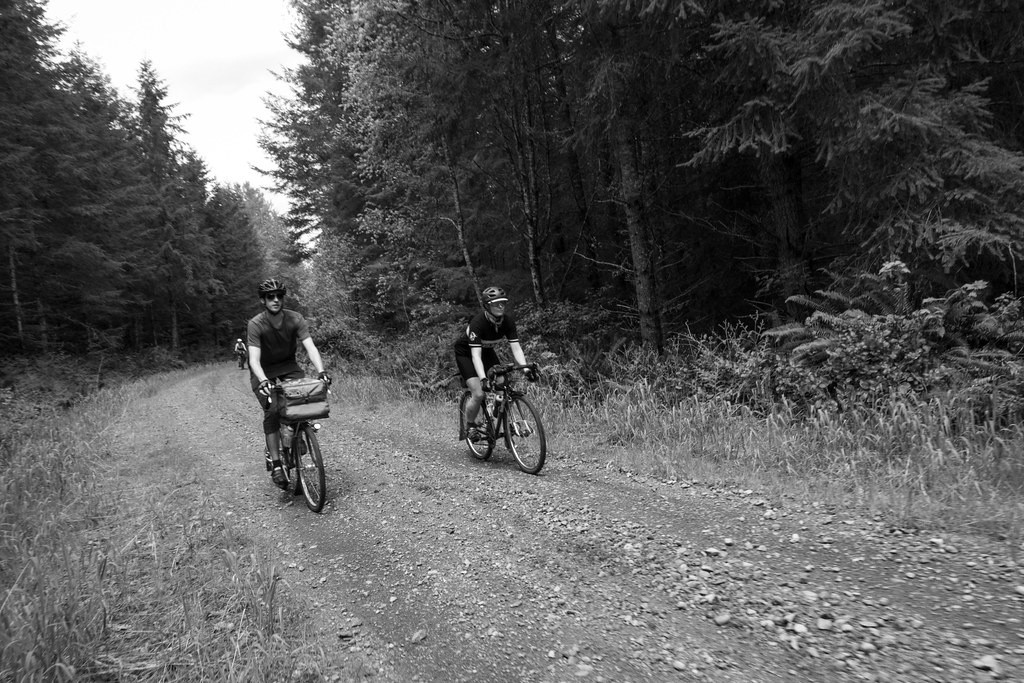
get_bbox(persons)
[246,279,333,486]
[453,287,539,449]
[234,338,247,367]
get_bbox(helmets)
[237,338,242,342]
[258,279,286,292]
[480,286,509,304]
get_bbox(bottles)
[493,395,503,418]
[282,426,293,448]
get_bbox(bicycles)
[234,350,247,369]
[458,362,546,475]
[264,379,333,513]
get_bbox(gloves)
[480,378,493,392]
[524,370,540,382]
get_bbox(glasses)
[491,301,506,307]
[266,293,282,299]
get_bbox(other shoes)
[465,422,480,442]
[301,439,307,455]
[271,467,287,485]
[238,362,241,367]
